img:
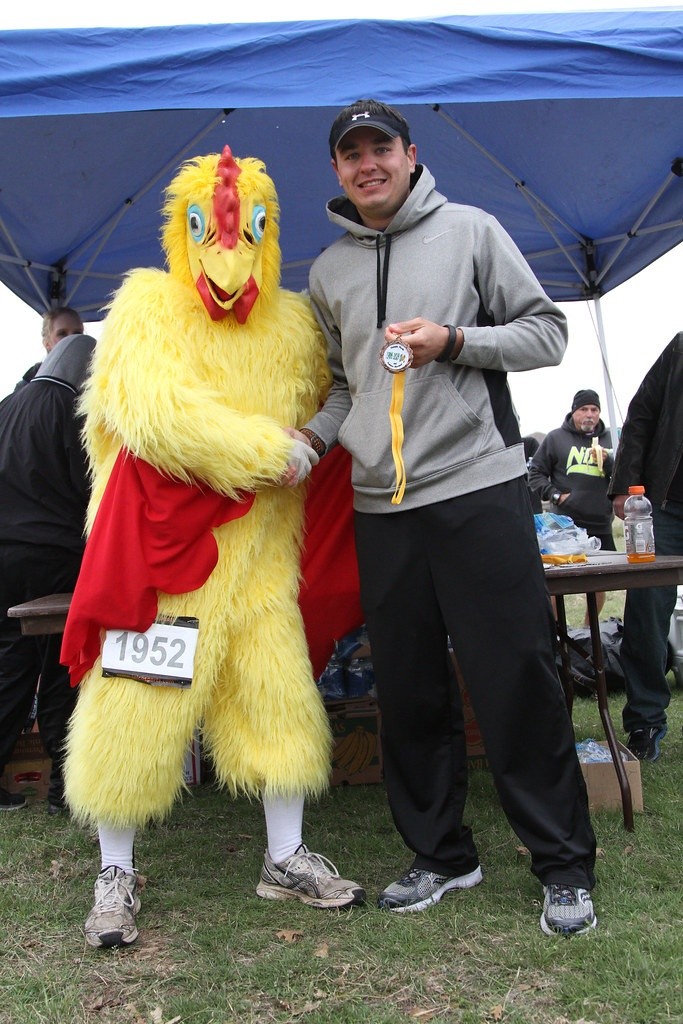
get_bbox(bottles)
[624,486,656,563]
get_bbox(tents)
[0,6,683,457]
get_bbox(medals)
[379,337,414,374]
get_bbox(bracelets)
[300,429,322,454]
[435,325,457,363]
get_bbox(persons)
[15,307,84,391]
[282,93,600,935]
[606,331,683,763]
[527,389,618,630]
[0,334,91,812]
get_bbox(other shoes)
[0,789,27,810]
[48,799,69,815]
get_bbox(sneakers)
[256,844,366,908]
[540,883,597,936]
[627,723,668,763]
[85,865,142,948]
[378,863,481,913]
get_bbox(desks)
[541,548,683,830]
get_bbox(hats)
[572,390,601,414]
[329,101,411,156]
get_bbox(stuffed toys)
[60,142,365,946]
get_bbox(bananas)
[585,437,603,472]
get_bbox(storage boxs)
[2,632,488,804]
[573,737,644,814]
